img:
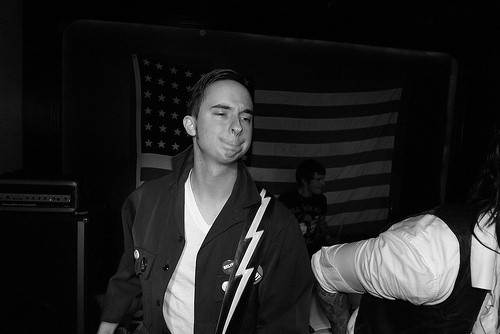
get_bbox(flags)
[126,48,405,253]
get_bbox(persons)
[309,148,500,333]
[96,68,313,334]
[273,158,334,251]
